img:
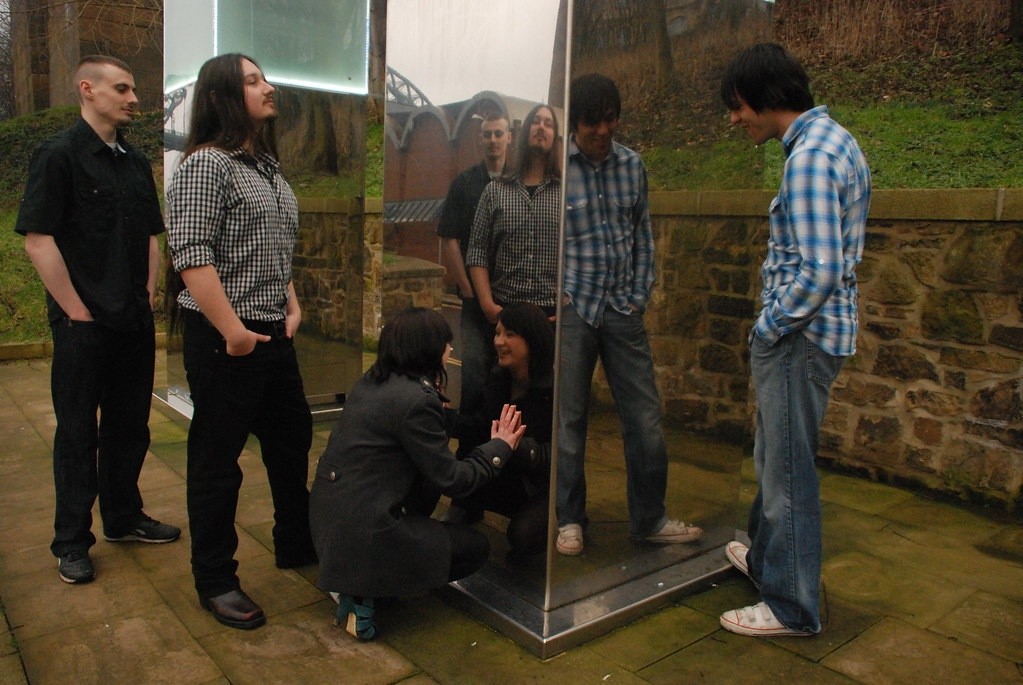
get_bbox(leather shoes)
[199,588,267,629]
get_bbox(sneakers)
[639,516,701,544]
[58,549,96,584]
[103,512,182,544]
[725,541,761,589]
[719,599,822,637]
[556,524,582,554]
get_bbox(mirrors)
[372,0,773,652]
[156,0,371,432]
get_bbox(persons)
[465,99,568,509]
[718,42,874,642]
[306,307,524,640]
[556,71,712,558]
[439,299,560,558]
[14,54,182,584]
[437,107,525,521]
[165,52,316,633]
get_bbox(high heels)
[331,593,375,643]
